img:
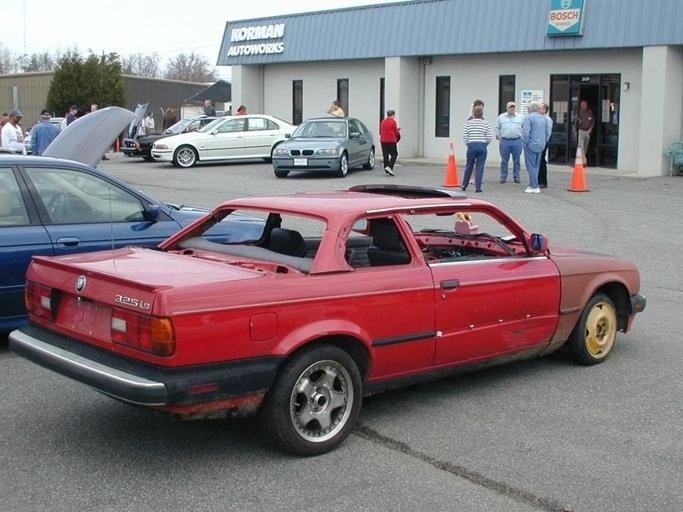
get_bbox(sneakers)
[385,166,393,176]
[524,183,547,193]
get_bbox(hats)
[10,110,23,117]
[506,101,516,108]
[41,109,52,116]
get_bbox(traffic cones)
[441,141,462,187]
[566,147,592,194]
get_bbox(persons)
[465,100,484,185]
[459,106,493,193]
[521,104,530,128]
[494,101,525,184]
[223,105,232,116]
[325,99,345,118]
[571,100,595,169]
[521,100,546,194]
[0,104,178,161]
[538,102,553,189]
[201,98,216,123]
[235,104,248,115]
[377,110,402,176]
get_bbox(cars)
[118,114,375,177]
[8,182,648,457]
[1,105,269,337]
[23,117,66,155]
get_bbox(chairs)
[367,220,410,266]
[266,227,306,259]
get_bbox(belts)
[501,137,520,140]
